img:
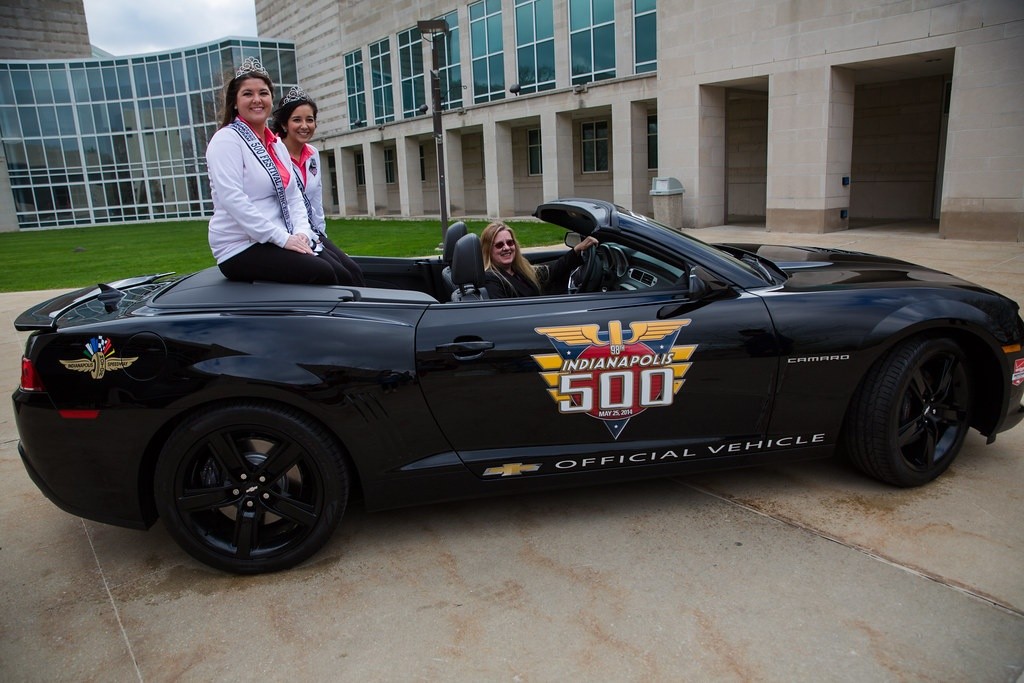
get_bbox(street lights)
[416,19,451,242]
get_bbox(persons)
[205,57,352,286]
[480,219,599,299]
[268,85,365,286]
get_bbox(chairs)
[440,221,490,302]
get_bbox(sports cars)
[10,199,1024,578]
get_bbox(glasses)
[492,240,514,249]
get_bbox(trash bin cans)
[648,175,686,231]
